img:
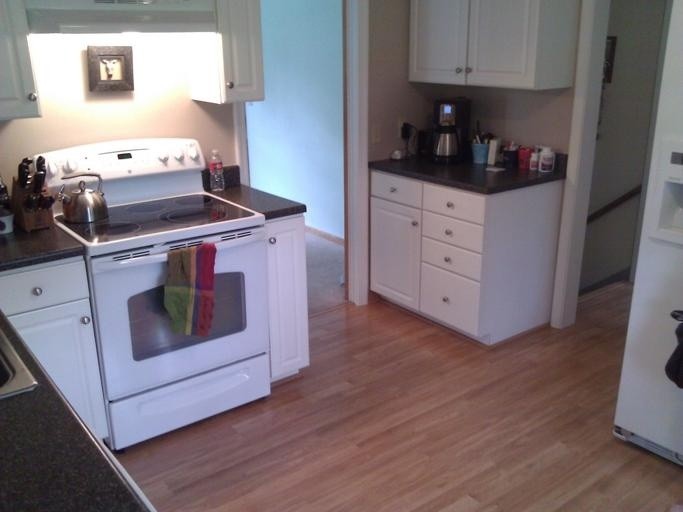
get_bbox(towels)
[162,242,217,338]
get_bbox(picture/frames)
[86,44,134,93]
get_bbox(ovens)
[87,226,269,402]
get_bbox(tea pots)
[57,171,108,224]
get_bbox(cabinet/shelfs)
[266,211,310,384]
[0,254,110,440]
[407,0,582,91]
[368,168,565,346]
[610,0,683,466]
[181,0,266,106]
[26,1,220,37]
[1,1,43,122]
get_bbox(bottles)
[208,149,226,192]
[517,145,556,172]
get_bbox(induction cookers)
[54,192,267,256]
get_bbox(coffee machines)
[432,98,472,164]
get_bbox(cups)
[502,150,517,167]
[472,143,487,165]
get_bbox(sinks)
[0,328,39,401]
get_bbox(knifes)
[18,156,55,212]
